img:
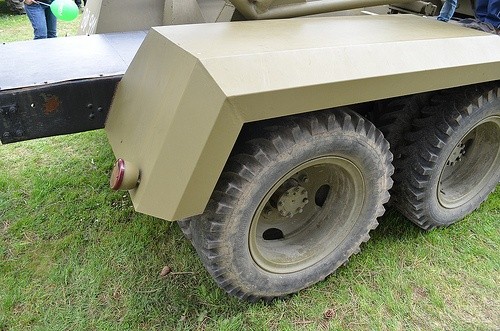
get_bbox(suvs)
[0,0,500,304]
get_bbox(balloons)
[48,0,79,22]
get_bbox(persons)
[18,0,59,39]
